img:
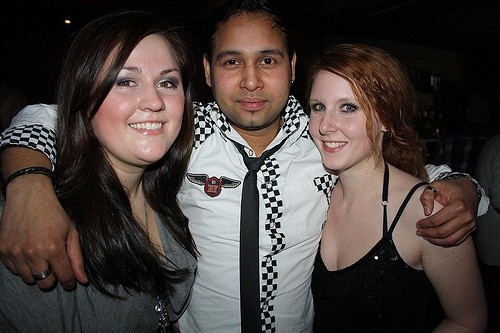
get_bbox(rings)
[30,264,53,283]
[423,186,438,199]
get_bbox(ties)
[226,137,288,333]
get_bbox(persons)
[0,2,500,332]
[0,13,198,332]
[307,37,490,332]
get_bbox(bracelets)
[1,166,61,198]
